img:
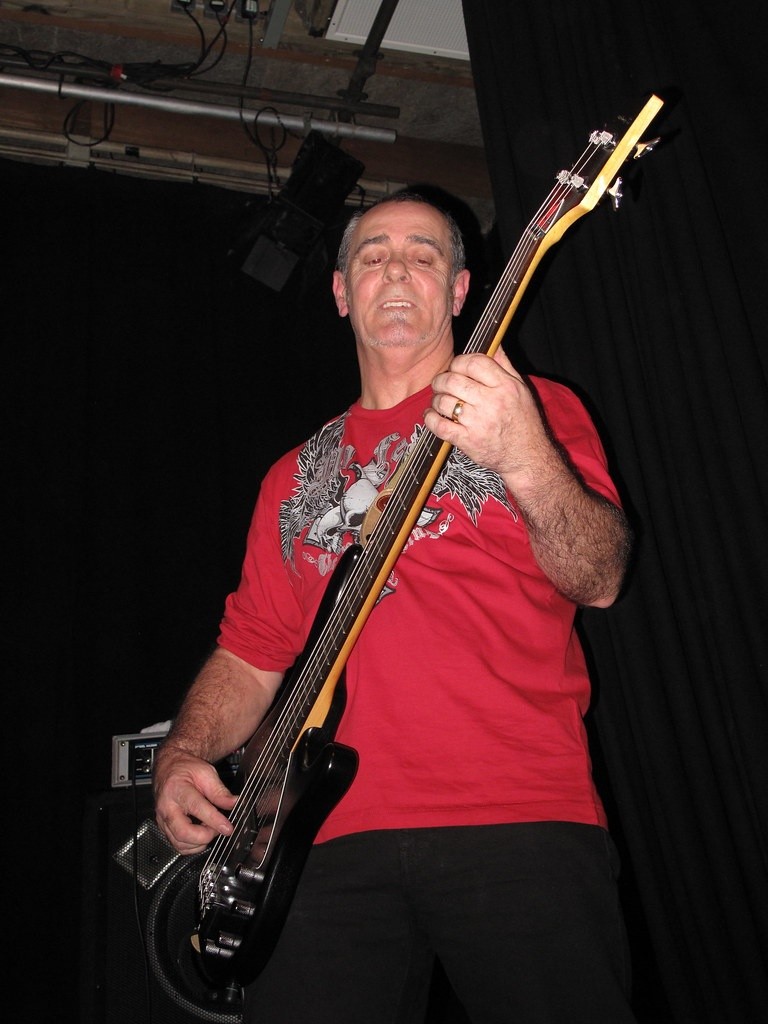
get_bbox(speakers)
[73,787,245,1024]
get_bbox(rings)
[452,401,464,423]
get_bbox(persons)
[151,192,628,1024]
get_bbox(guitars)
[184,81,666,1008]
